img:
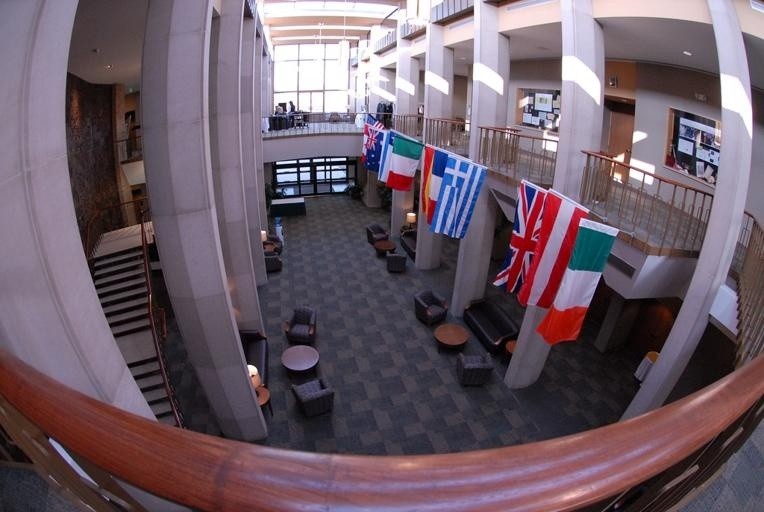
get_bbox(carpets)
[294,182,335,194]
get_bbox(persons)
[274,101,295,117]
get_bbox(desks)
[263,244,275,252]
[270,198,306,216]
[342,115,356,123]
[505,339,516,359]
[434,323,468,355]
[255,386,274,416]
[267,113,289,131]
[400,226,415,233]
[290,112,309,128]
[373,240,396,258]
[281,345,320,377]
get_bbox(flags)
[536,219,619,344]
[516,190,590,309]
[492,181,548,294]
[421,144,472,225]
[361,113,424,191]
[429,155,488,239]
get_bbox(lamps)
[261,230,267,242]
[246,364,260,397]
[406,213,415,229]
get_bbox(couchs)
[238,328,269,388]
[401,230,417,261]
[462,298,520,358]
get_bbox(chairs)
[293,114,305,128]
[455,352,493,387]
[285,305,318,345]
[264,252,282,272]
[366,222,389,246]
[290,377,334,421]
[413,288,448,329]
[263,235,282,254]
[385,252,407,274]
[329,112,340,122]
